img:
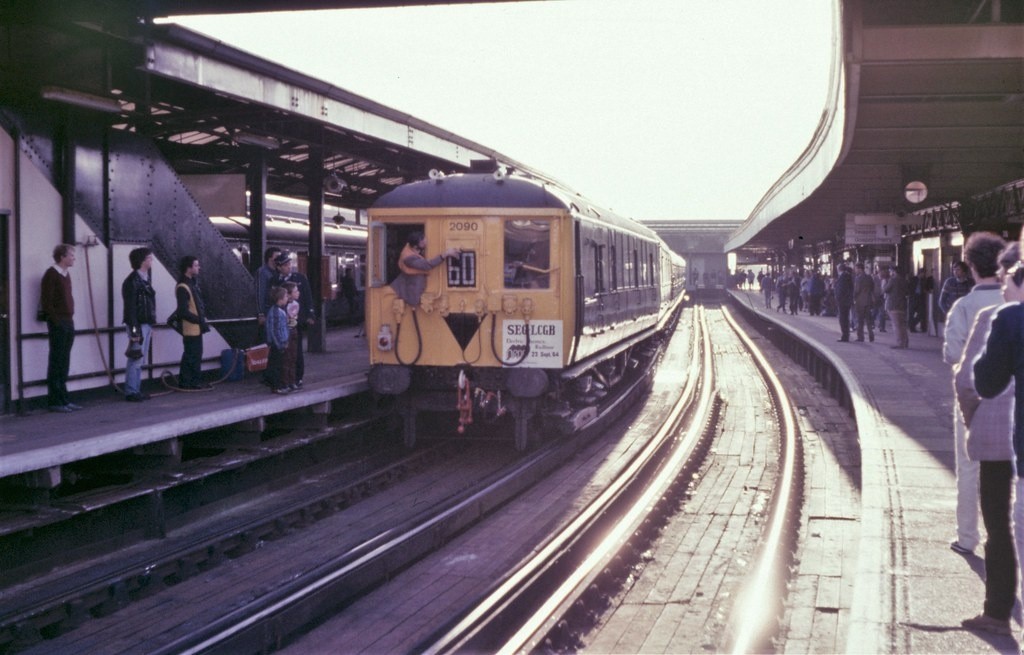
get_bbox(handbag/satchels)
[36,306,45,321]
[123,340,142,358]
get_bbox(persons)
[389,231,461,307]
[175,256,210,389]
[41,245,83,412]
[122,247,156,402]
[251,247,315,394]
[691,232,1024,635]
[336,267,363,325]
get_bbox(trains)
[364,157,692,457]
[208,209,367,328]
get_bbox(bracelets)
[440,255,444,261]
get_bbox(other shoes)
[275,379,305,394]
[182,383,212,391]
[890,343,907,350]
[49,402,80,413]
[125,390,150,403]
[836,336,846,339]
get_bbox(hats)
[273,253,290,263]
